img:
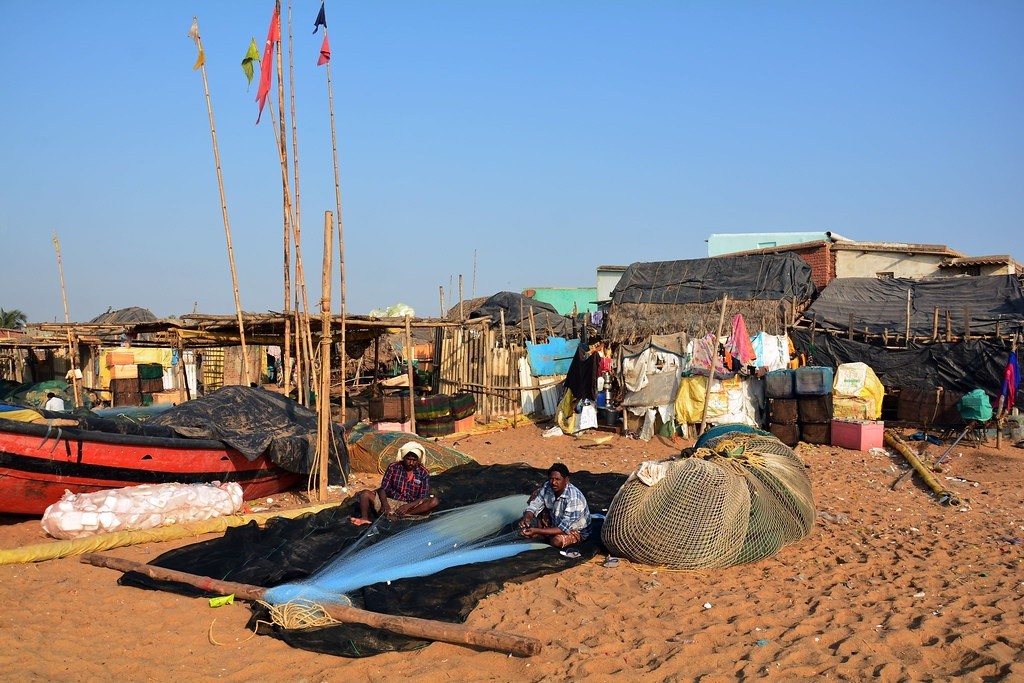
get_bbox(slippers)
[603,557,620,568]
[560,548,581,558]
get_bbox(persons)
[518,463,593,548]
[45,392,64,411]
[350,441,440,527]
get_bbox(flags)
[241,38,260,90]
[255,6,280,125]
[316,34,330,67]
[312,3,327,35]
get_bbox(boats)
[0,403,307,513]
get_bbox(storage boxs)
[831,417,884,450]
[455,415,474,433]
[106,352,180,407]
[369,396,410,423]
